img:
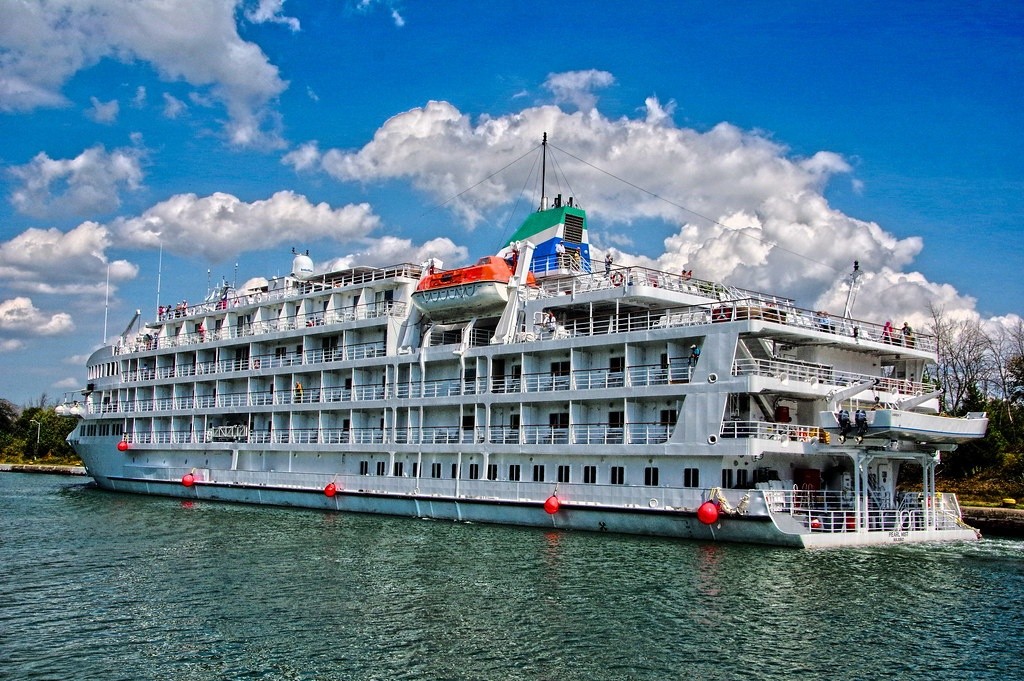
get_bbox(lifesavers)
[306,321,313,328]
[612,272,623,286]
[254,359,260,369]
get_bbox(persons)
[605,254,613,274]
[159,300,187,321]
[880,322,894,345]
[690,344,700,364]
[543,311,556,332]
[813,311,836,334]
[900,322,915,349]
[556,240,565,269]
[198,322,205,343]
[679,269,690,286]
[574,247,580,270]
[296,381,304,403]
[153,332,158,349]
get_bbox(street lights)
[30,419,40,443]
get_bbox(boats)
[66,133,987,552]
